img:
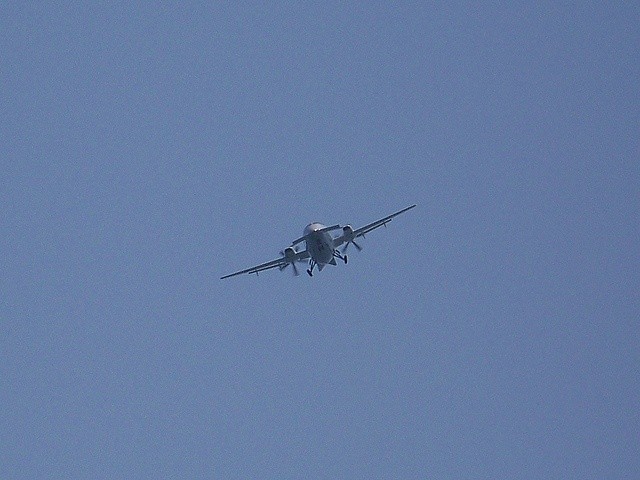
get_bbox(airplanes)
[220,202,418,279]
